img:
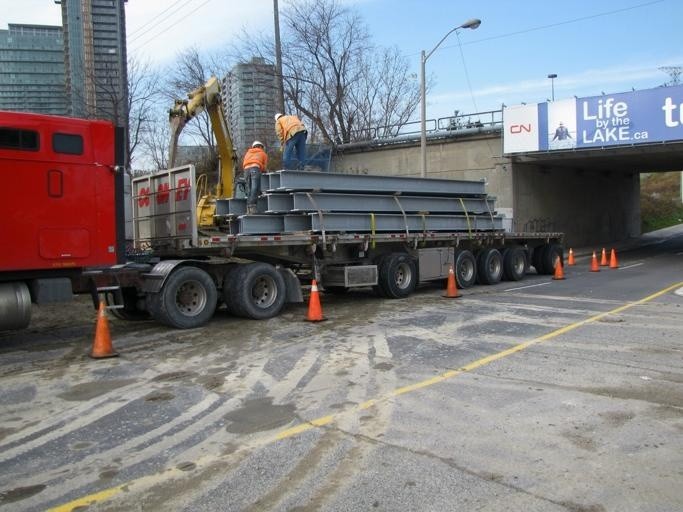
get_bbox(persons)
[242,140,273,216]
[274,113,308,171]
[550,119,574,141]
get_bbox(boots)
[246,204,261,214]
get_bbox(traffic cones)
[303,276,329,324]
[440,263,466,299]
[609,248,620,269]
[549,255,566,280]
[88,300,121,362]
[590,249,601,273]
[567,247,576,266]
[599,247,609,268]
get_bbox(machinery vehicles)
[161,73,241,237]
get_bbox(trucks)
[0,105,565,335]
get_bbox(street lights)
[549,74,559,103]
[417,17,481,178]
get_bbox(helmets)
[252,140,264,148]
[274,113,283,121]
